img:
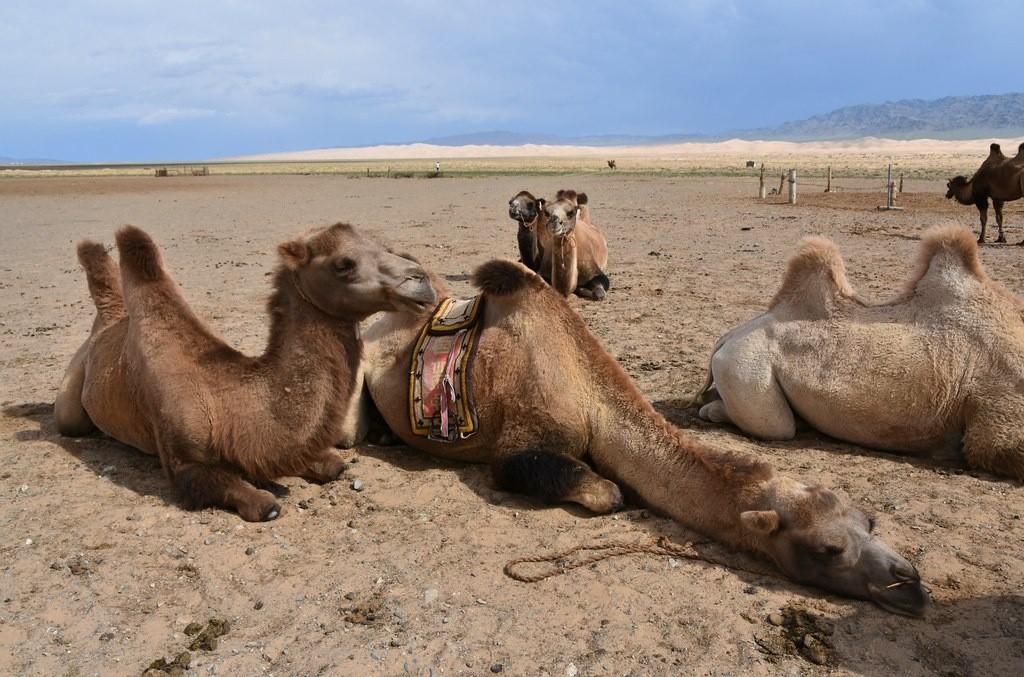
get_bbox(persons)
[436,162,440,170]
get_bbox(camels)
[941,143,1024,248]
[505,190,610,303]
[608,159,617,170]
[665,220,1024,505]
[48,218,437,523]
[330,257,929,618]
